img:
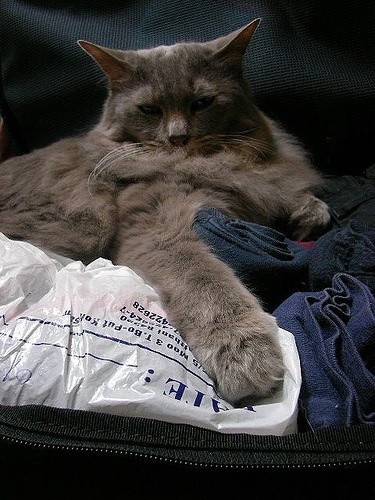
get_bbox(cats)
[0,17,335,409]
